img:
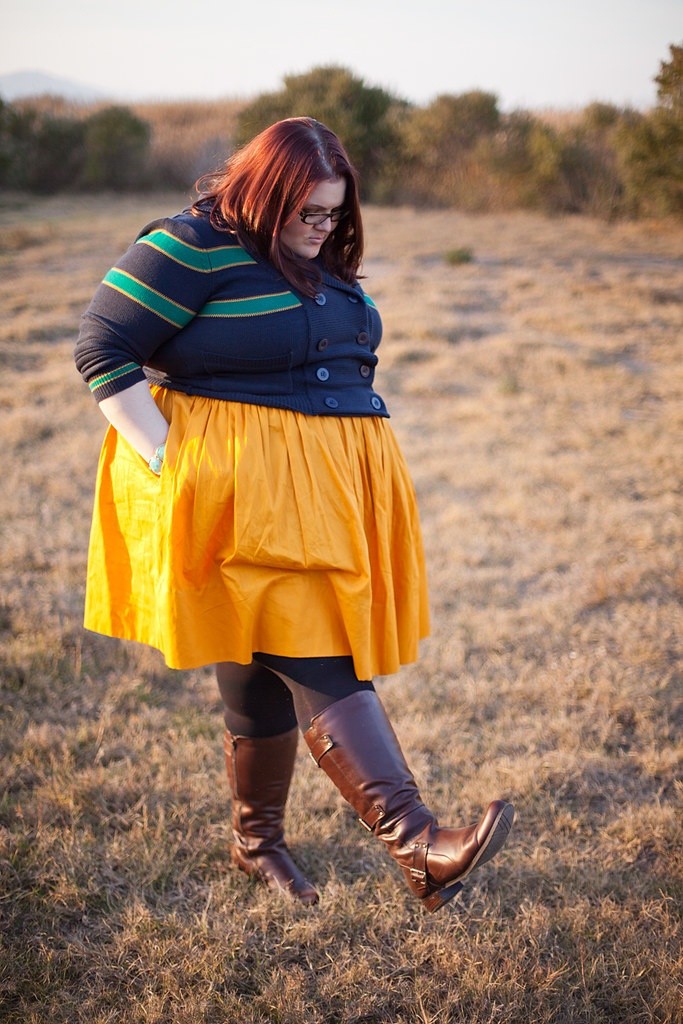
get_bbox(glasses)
[297,208,351,225]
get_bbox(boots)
[301,689,514,913]
[222,724,318,908]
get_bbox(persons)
[73,116,514,915]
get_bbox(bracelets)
[149,443,166,473]
